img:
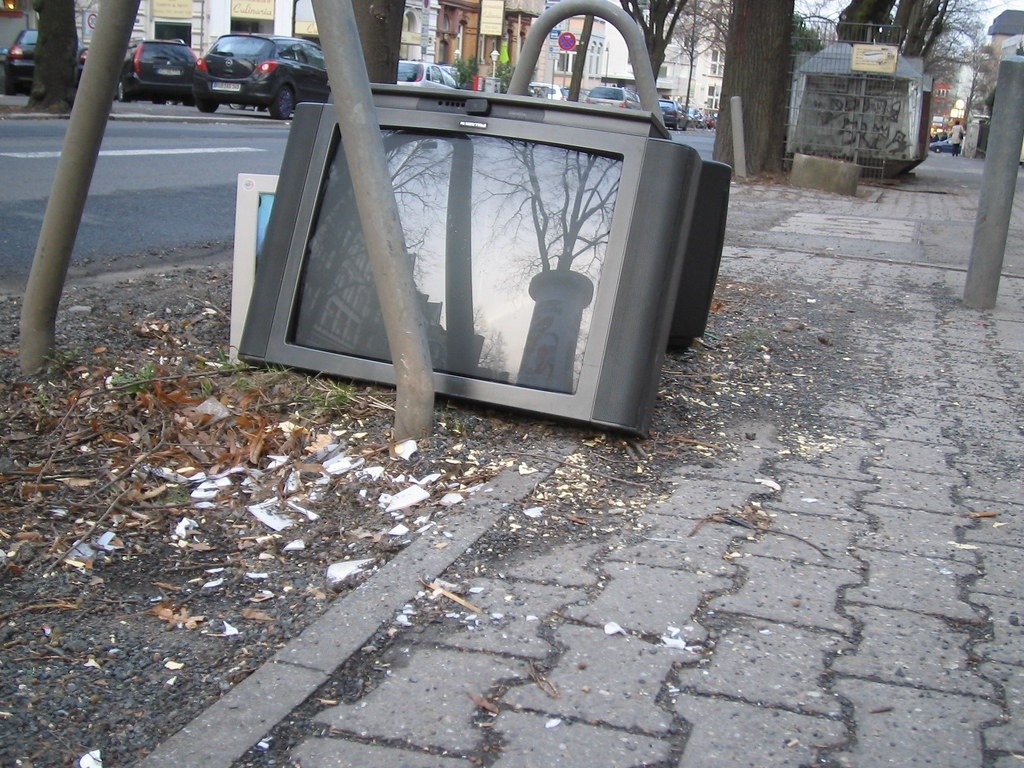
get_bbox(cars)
[396,60,457,91]
[194,31,329,119]
[688,108,705,128]
[929,137,963,154]
[527,82,564,101]
[658,99,688,131]
[585,87,641,110]
[1,29,84,95]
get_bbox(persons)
[950,120,966,156]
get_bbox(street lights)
[388,142,438,186]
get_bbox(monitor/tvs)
[238,80,702,437]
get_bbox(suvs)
[112,37,200,109]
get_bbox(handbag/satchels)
[959,132,963,140]
